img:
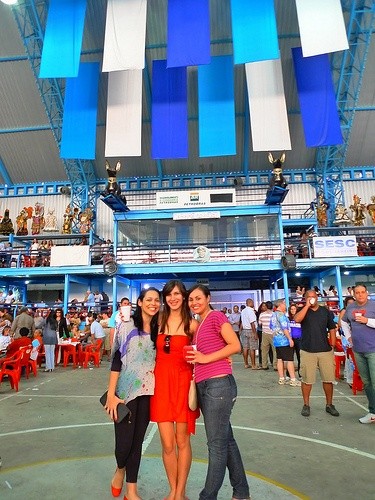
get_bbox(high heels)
[43,369,54,372]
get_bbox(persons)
[182,285,254,500]
[149,280,200,500]
[0,235,117,269]
[0,288,132,372]
[220,283,375,424]
[104,286,162,500]
[281,228,375,261]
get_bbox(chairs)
[328,336,364,396]
[0,339,103,392]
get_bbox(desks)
[55,343,83,368]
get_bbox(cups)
[183,345,197,362]
[120,306,131,321]
[355,313,362,322]
[68,339,71,343]
[310,298,315,304]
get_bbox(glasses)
[163,335,172,354]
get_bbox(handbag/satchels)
[188,381,198,411]
[100,389,132,424]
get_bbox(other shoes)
[301,404,310,416]
[274,369,278,371]
[326,404,339,416]
[359,413,375,424]
[111,468,125,497]
[248,365,262,370]
[278,379,289,385]
[289,378,301,387]
[262,367,269,370]
[123,495,128,500]
